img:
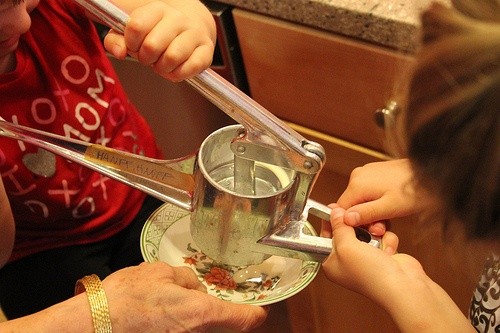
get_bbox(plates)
[139,203,321,307]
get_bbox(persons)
[320,0,500,333]
[0,176,270,333]
[0,0,217,321]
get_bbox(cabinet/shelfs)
[232,8,490,333]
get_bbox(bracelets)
[76,274,112,333]
